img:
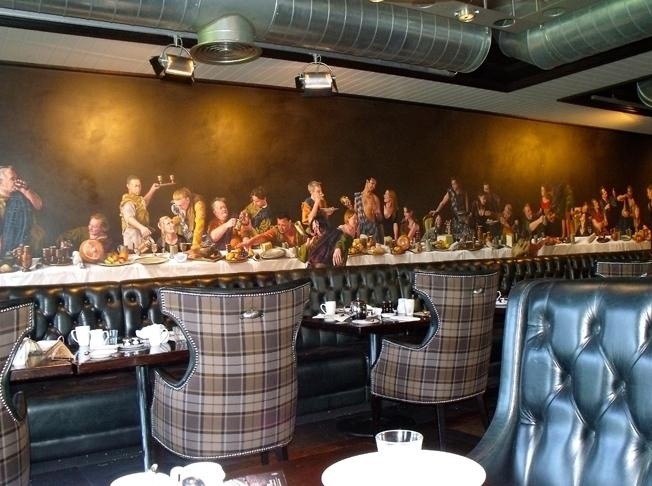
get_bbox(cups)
[2,237,73,273]
[318,297,428,325]
[99,236,309,266]
[70,325,186,363]
[109,461,226,486]
[374,429,427,461]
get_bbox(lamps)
[295,52,340,100]
[150,34,198,85]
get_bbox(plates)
[319,448,488,485]
[319,206,338,213]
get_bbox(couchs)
[1,253,652,486]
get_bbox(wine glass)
[156,174,177,184]
